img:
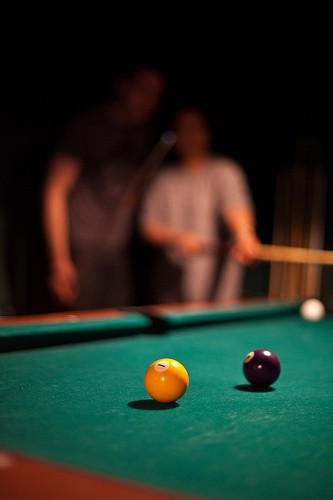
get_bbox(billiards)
[300,298,326,323]
[238,349,281,389]
[144,358,189,403]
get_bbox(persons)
[42,60,169,312]
[139,104,259,299]
